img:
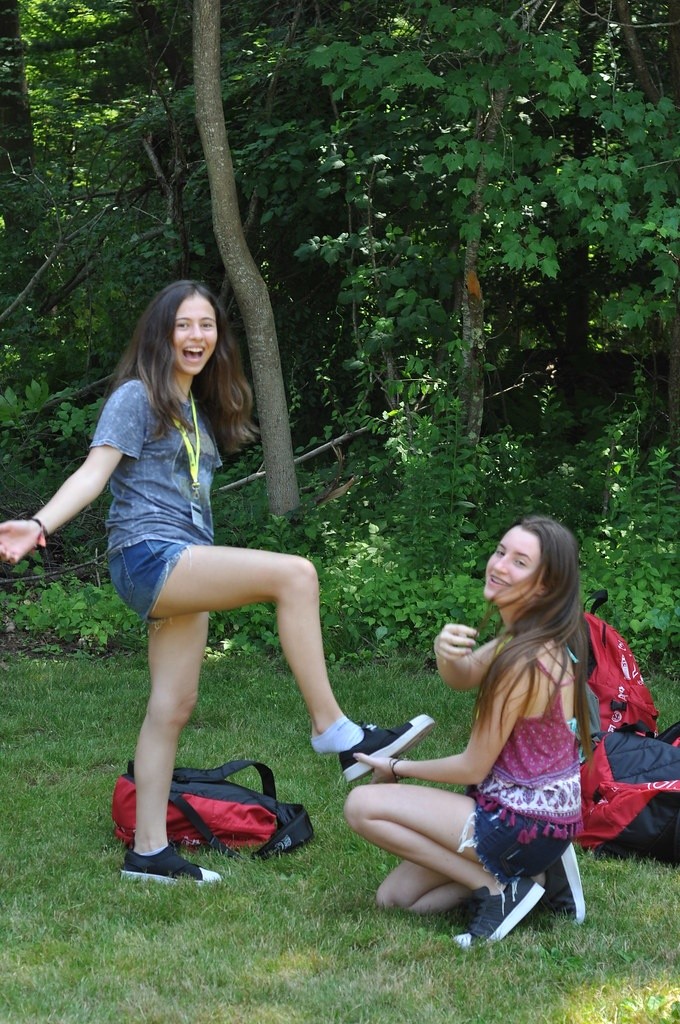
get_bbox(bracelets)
[389,758,404,782]
[29,518,48,547]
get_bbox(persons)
[345,516,587,946]
[0,280,435,886]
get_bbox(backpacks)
[581,613,660,739]
[580,719,680,867]
[115,760,313,864]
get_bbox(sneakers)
[339,714,435,783]
[120,840,221,887]
[452,876,545,951]
[538,841,585,924]
